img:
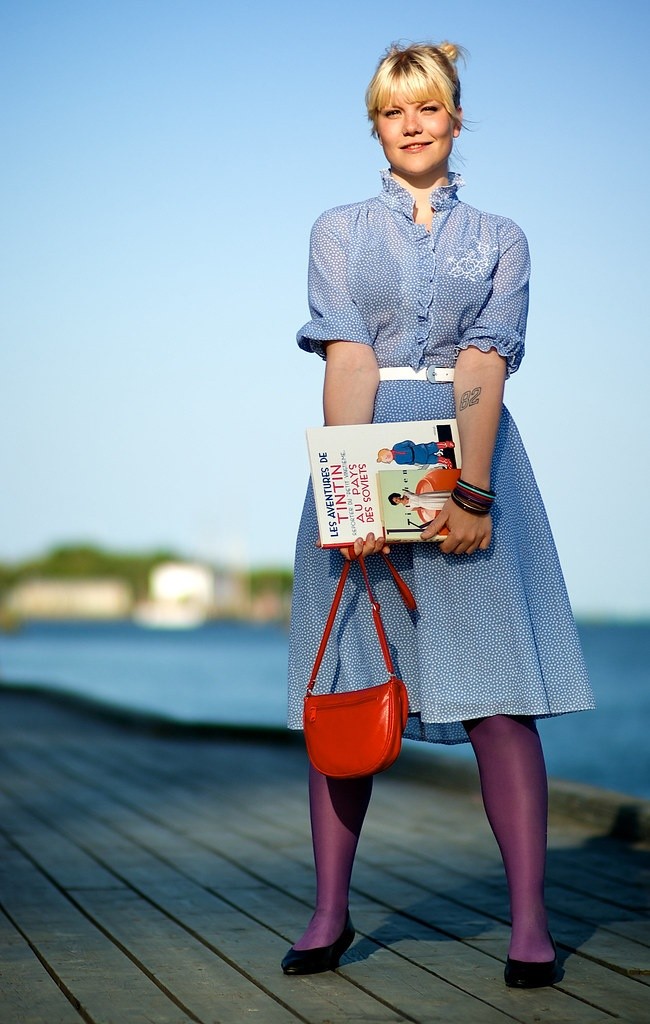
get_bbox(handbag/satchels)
[303,544,417,778]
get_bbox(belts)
[378,367,455,382]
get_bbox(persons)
[387,489,451,512]
[282,42,605,991]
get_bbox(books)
[306,418,461,549]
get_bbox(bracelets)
[452,479,496,516]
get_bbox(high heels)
[281,909,355,975]
[504,930,558,988]
